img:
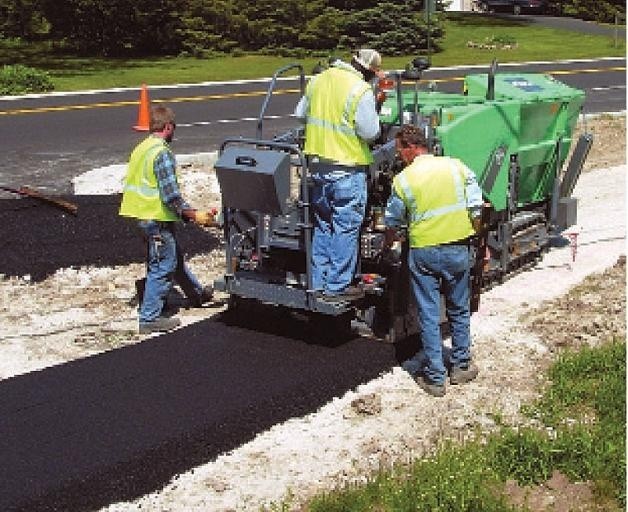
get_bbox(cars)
[484,0,549,15]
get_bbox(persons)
[296,47,384,304]
[385,123,484,399]
[117,106,216,334]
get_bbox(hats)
[354,48,386,79]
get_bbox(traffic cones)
[132,84,151,132]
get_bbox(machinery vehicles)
[211,58,592,344]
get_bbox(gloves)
[193,210,216,227]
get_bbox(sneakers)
[448,366,480,385]
[189,284,215,308]
[137,317,183,335]
[414,370,447,397]
[316,285,368,304]
